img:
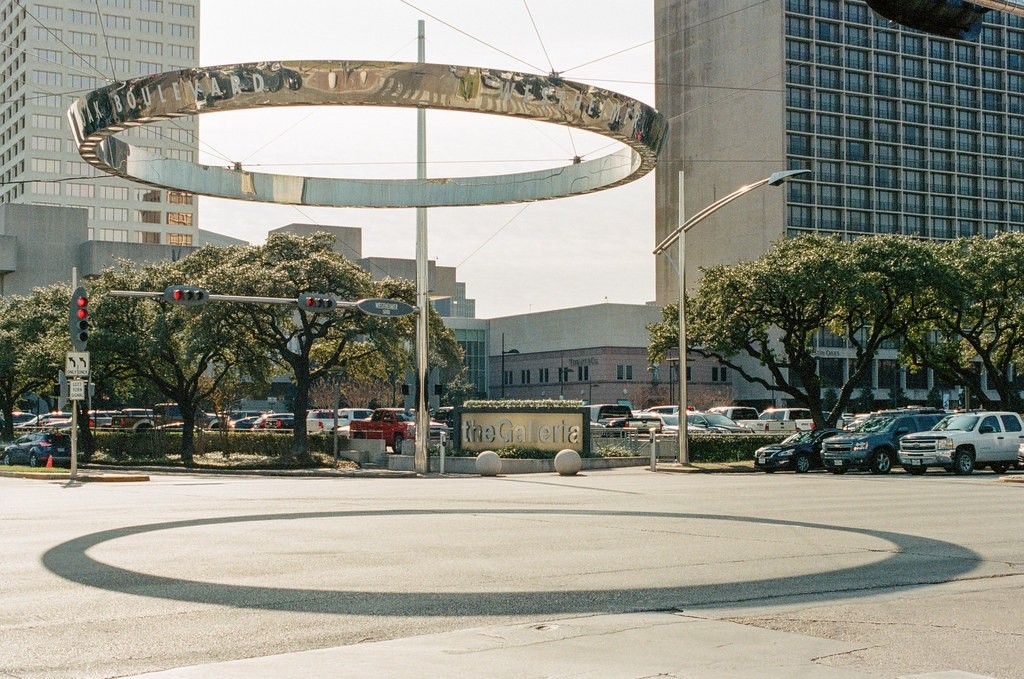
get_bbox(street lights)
[653,168,814,468]
[588,377,600,405]
[501,332,521,401]
[560,367,574,400]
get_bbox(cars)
[428,406,454,438]
[753,428,852,474]
[587,405,945,436]
[1,431,72,468]
[0,402,374,438]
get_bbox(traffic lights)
[298,293,338,313]
[68,286,89,353]
[164,284,209,306]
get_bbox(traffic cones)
[45,453,53,469]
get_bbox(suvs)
[820,412,954,475]
[898,412,1024,476]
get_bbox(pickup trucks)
[348,408,451,453]
